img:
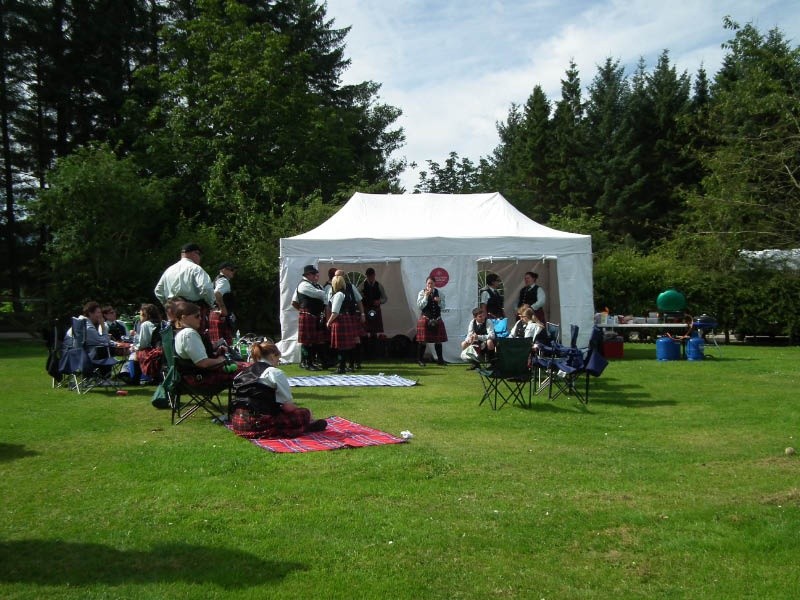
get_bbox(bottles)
[224,358,237,373]
[240,340,248,362]
[601,308,609,324]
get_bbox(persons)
[99,306,130,347]
[164,296,184,337]
[232,341,328,440]
[416,276,448,366]
[67,301,108,381]
[209,261,239,346]
[461,307,497,371]
[119,303,162,385]
[508,304,552,382]
[516,272,547,336]
[292,265,365,374]
[357,268,387,339]
[154,244,215,306]
[174,302,251,423]
[481,274,507,319]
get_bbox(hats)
[365,268,375,275]
[181,244,200,253]
[302,265,317,276]
[219,261,233,269]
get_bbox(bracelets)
[360,313,364,316]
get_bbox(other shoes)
[111,371,131,381]
[418,360,426,366]
[306,419,327,433]
[98,378,118,386]
[467,364,481,371]
[300,358,360,374]
[438,361,447,366]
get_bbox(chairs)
[548,326,608,404]
[46,318,101,388]
[545,322,561,345]
[474,336,534,410]
[159,326,233,427]
[533,324,580,394]
[70,317,127,395]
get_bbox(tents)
[275,192,595,363]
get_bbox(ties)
[111,323,115,325]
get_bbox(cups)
[233,338,238,346]
[614,316,618,324]
[135,335,139,344]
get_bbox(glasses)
[106,311,114,315]
[186,313,201,319]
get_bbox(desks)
[597,322,722,359]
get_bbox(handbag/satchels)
[367,310,376,320]
[492,317,509,337]
[427,319,438,332]
